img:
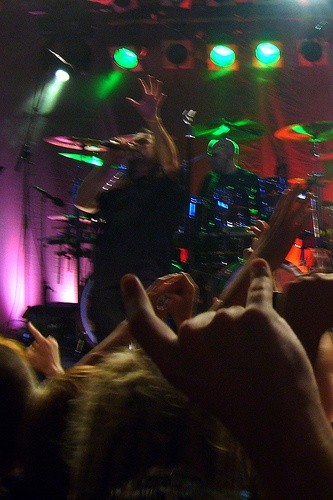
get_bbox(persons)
[0,182,333,500]
[191,137,277,314]
[72,75,191,344]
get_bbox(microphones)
[103,138,135,150]
[32,185,65,208]
[46,47,72,72]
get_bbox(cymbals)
[60,152,103,167]
[49,215,92,226]
[42,134,150,153]
[191,116,271,141]
[274,120,333,142]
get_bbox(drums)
[257,175,288,219]
[196,185,263,239]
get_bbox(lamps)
[48,40,322,83]
